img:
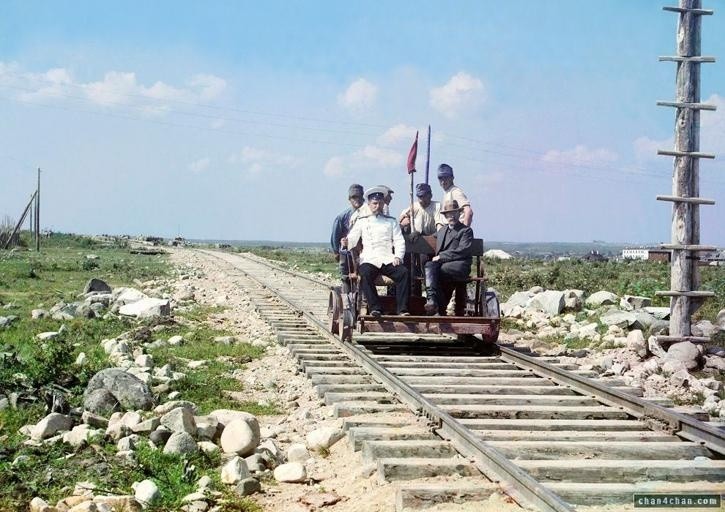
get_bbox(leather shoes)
[372,310,380,316]
[424,300,438,312]
[400,311,409,316]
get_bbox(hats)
[440,200,464,214]
[348,183,363,198]
[364,186,395,200]
[415,184,431,197]
[438,165,454,178]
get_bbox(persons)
[409,200,474,314]
[329,184,365,290]
[338,186,409,317]
[398,182,440,298]
[433,163,473,315]
[349,185,395,230]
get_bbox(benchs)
[345,234,489,317]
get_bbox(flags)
[406,138,418,173]
